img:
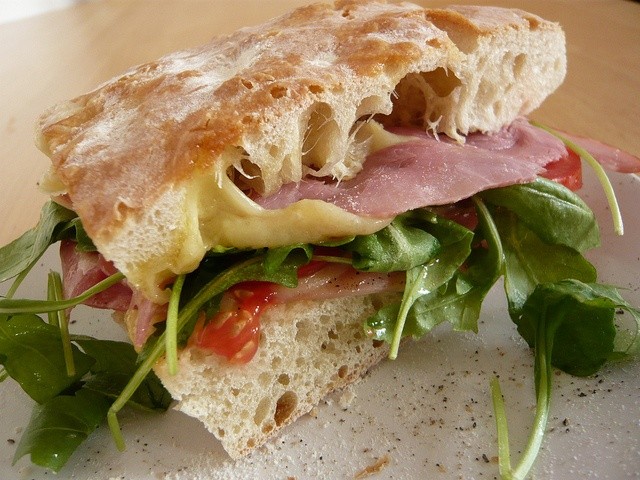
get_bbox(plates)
[1,146,639,479]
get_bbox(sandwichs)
[31,0,637,460]
